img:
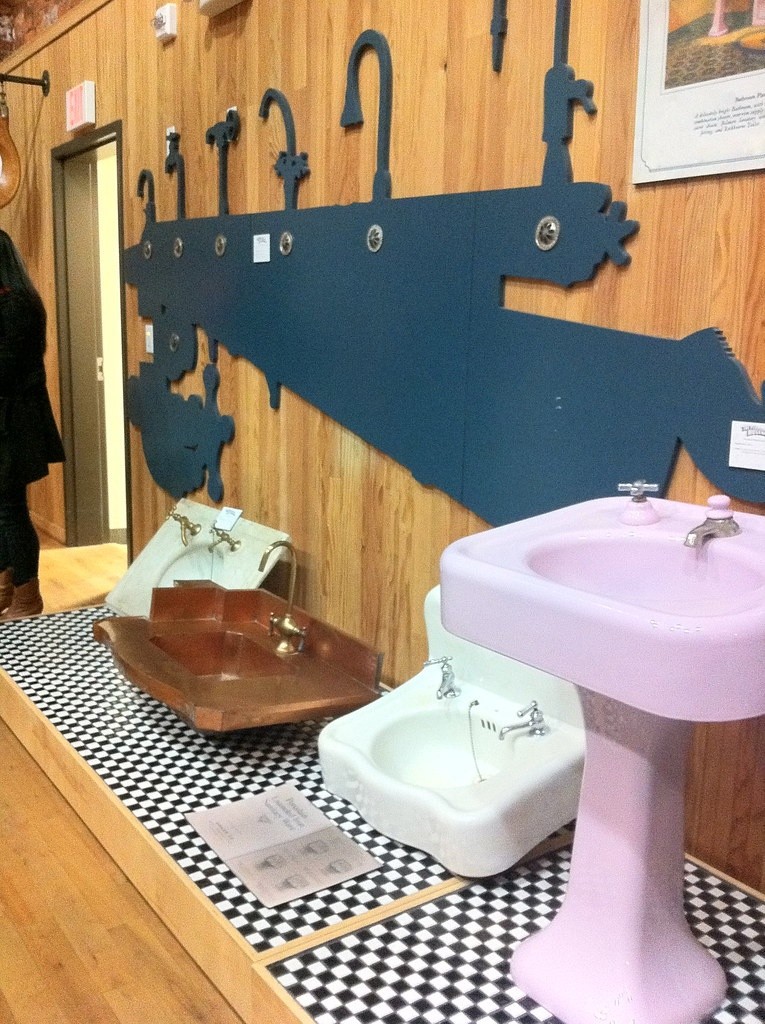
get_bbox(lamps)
[0,69,51,210]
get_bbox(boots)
[0,579,44,619]
[0,568,14,611]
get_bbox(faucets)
[423,655,462,700]
[682,493,742,548]
[498,699,550,739]
[258,540,309,658]
[207,518,242,554]
[164,504,202,548]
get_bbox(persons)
[0,230,65,619]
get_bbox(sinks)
[317,665,585,878]
[105,497,292,617]
[92,614,378,739]
[440,496,765,722]
